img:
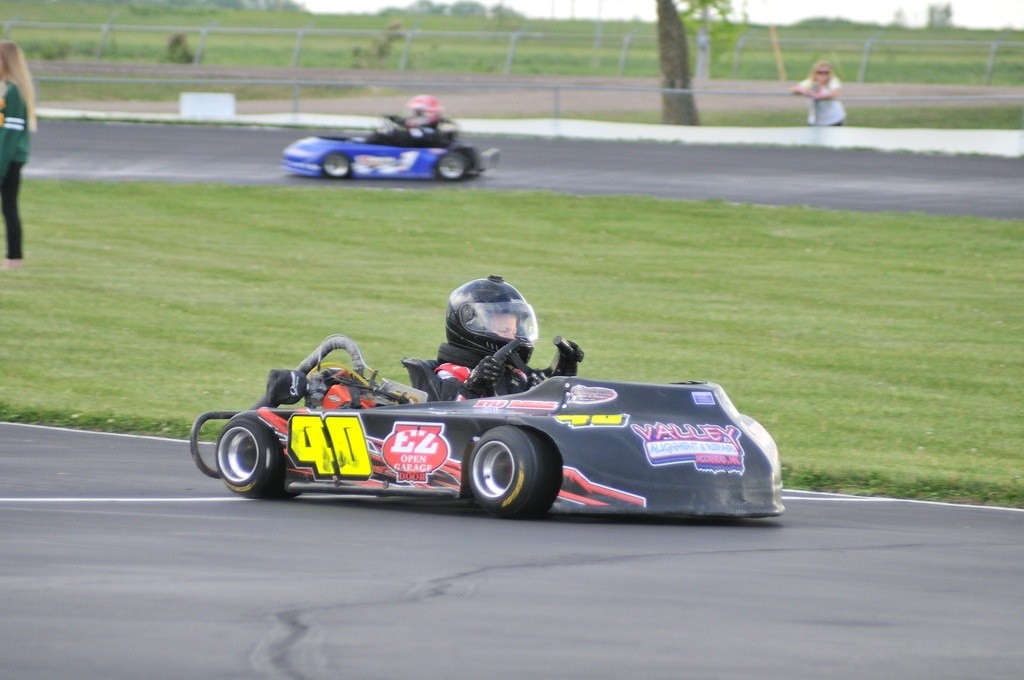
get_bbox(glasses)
[815,71,830,75]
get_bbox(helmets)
[447,274,539,364]
[406,93,442,127]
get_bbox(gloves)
[528,372,547,388]
[464,356,499,389]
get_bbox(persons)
[0,40,37,267]
[434,276,583,401]
[367,97,440,147]
[793,62,846,126]
